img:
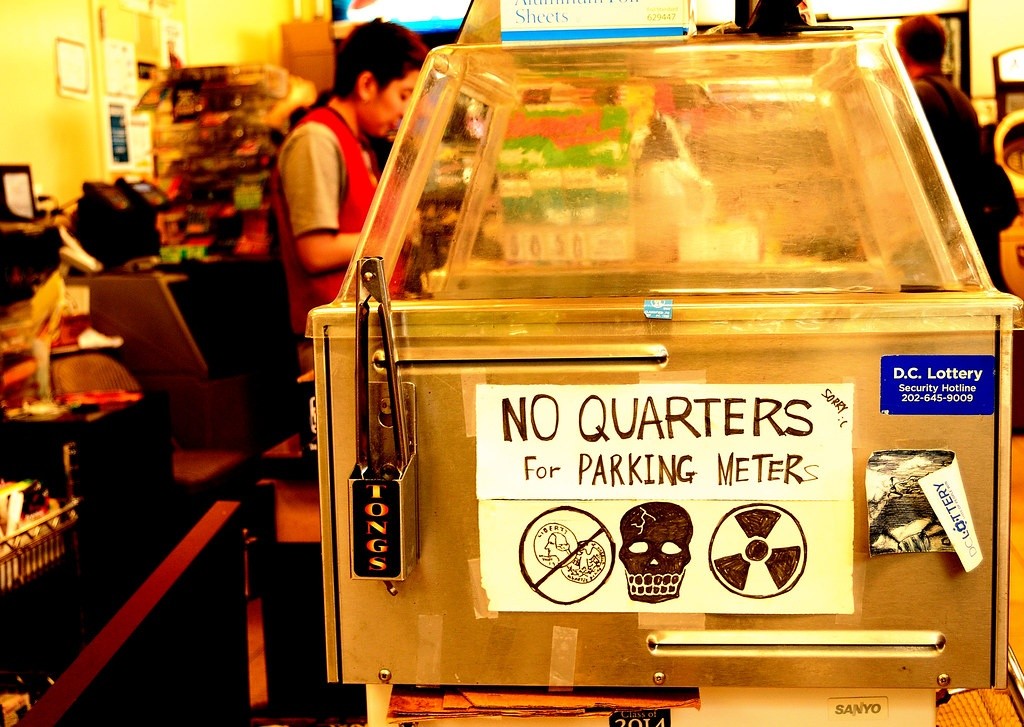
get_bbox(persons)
[273,18,430,489]
[895,14,1019,292]
